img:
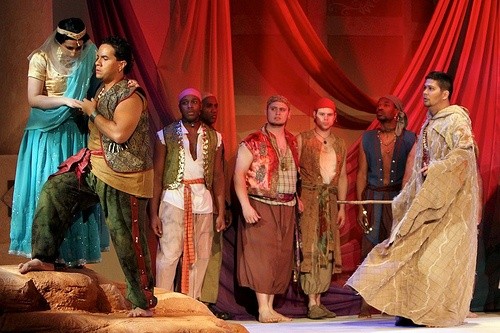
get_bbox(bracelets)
[88,111,99,123]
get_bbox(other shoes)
[307,304,337,319]
[394,315,427,326]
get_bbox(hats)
[201,90,217,100]
[313,96,336,113]
[377,93,405,113]
[267,94,291,108]
[177,87,201,103]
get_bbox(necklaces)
[314,127,331,144]
[377,127,399,155]
[187,120,195,125]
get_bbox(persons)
[18,35,158,317]
[343,71,482,328]
[198,91,237,318]
[296,96,348,320]
[150,88,226,298]
[233,94,303,324]
[356,95,420,318]
[7,17,138,268]
